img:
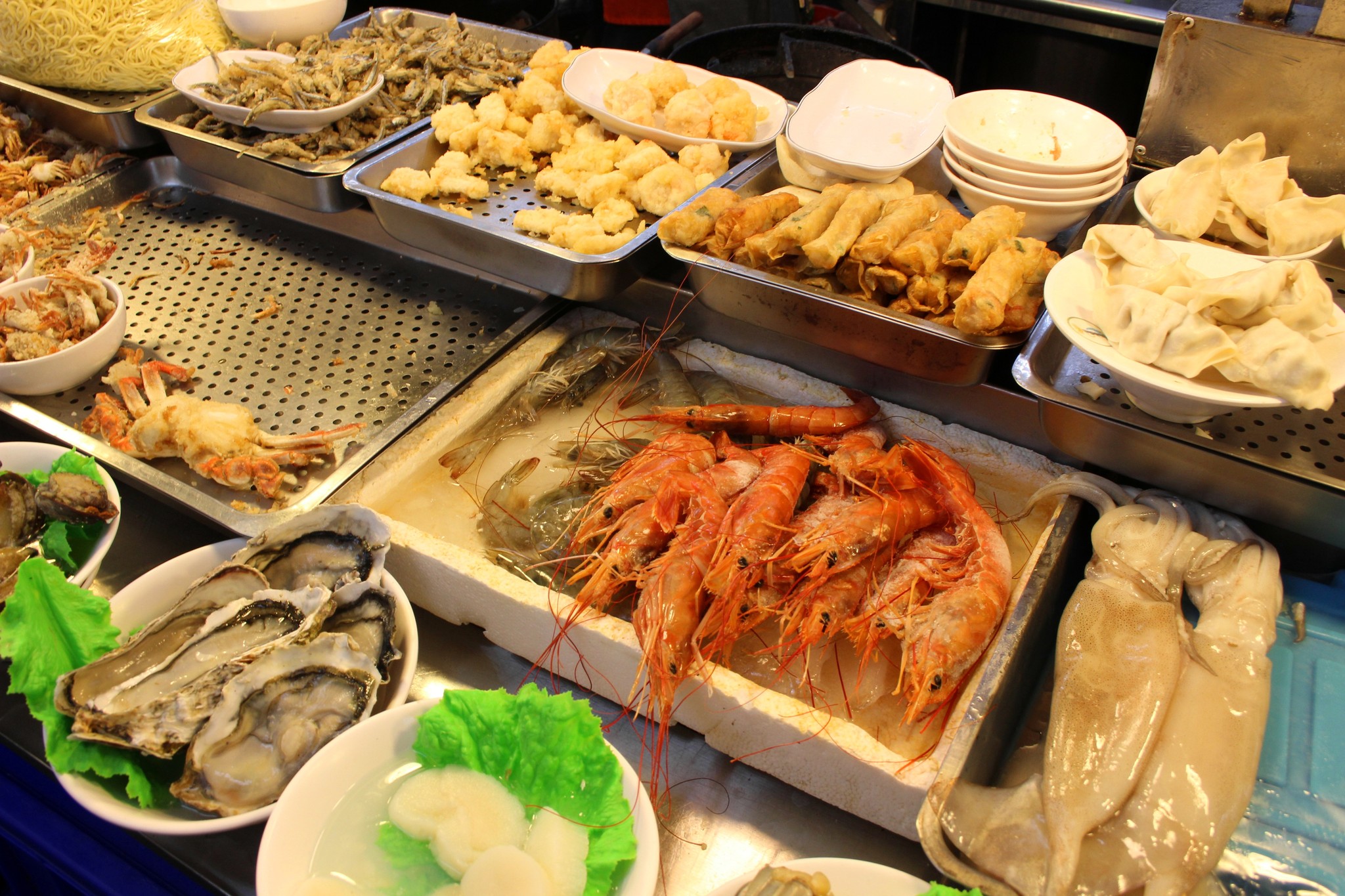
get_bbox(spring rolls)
[654,176,1061,337]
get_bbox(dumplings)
[1081,133,1345,410]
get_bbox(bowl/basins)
[0,442,121,591]
[706,857,932,896]
[1043,239,1345,424]
[940,89,1128,242]
[786,59,954,183]
[42,537,420,835]
[0,273,127,396]
[216,0,348,48]
[0,223,35,289]
[256,698,660,896]
[172,50,384,134]
[1134,167,1332,262]
[561,47,788,153]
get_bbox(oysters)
[46,497,407,819]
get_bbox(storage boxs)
[0,0,1345,896]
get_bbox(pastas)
[0,0,233,93]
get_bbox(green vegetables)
[377,683,641,896]
[0,450,190,815]
[910,881,985,896]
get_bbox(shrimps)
[436,310,1019,821]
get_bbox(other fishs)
[923,457,1315,896]
[166,4,537,165]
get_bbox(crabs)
[77,352,372,497]
[0,221,120,364]
[0,100,137,219]
